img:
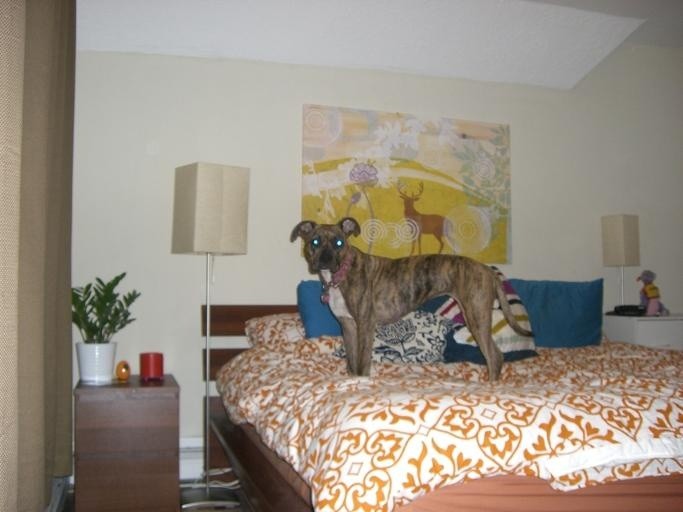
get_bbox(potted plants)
[72,271,141,385]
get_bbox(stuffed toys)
[635,269,669,316]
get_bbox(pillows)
[329,311,445,364]
[298,264,527,361]
[244,312,304,347]
[509,278,602,347]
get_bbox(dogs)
[288,217,536,382]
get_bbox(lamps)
[601,214,641,315]
[170,161,250,511]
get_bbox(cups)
[140,353,164,383]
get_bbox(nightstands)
[602,314,683,353]
[73,374,182,512]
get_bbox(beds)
[201,305,682,511]
